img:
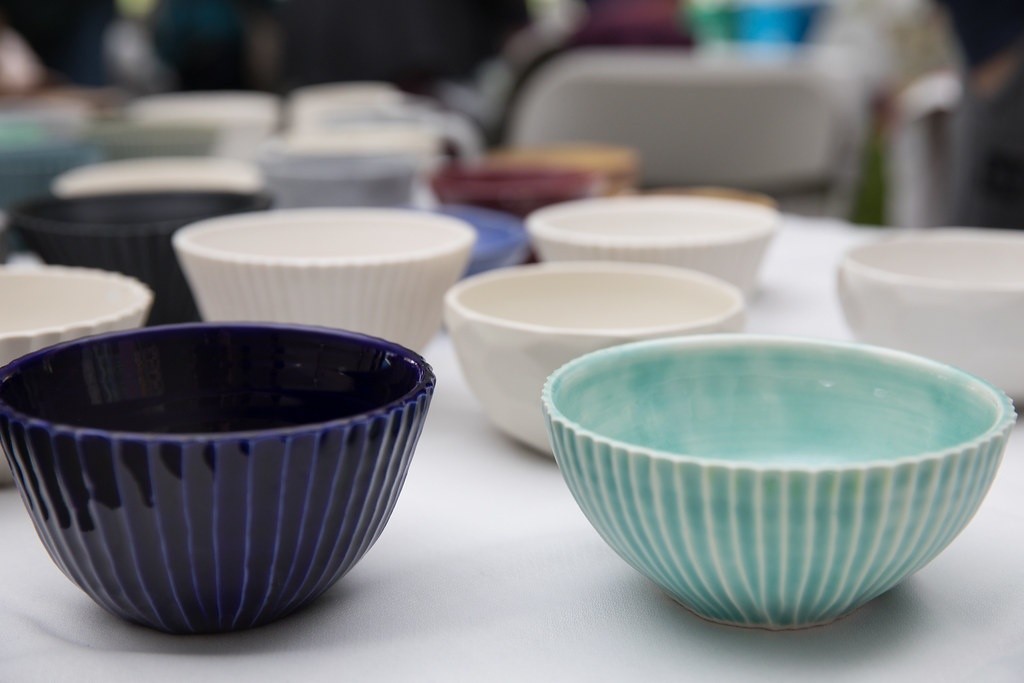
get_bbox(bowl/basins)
[170,206,478,354]
[521,193,782,285]
[541,333,1018,629]
[441,261,747,459]
[833,227,1024,409]
[16,191,274,328]
[0,263,154,367]
[1,320,436,636]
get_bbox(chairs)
[881,73,980,228]
[502,49,864,220]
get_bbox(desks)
[0,212,1024,683]
